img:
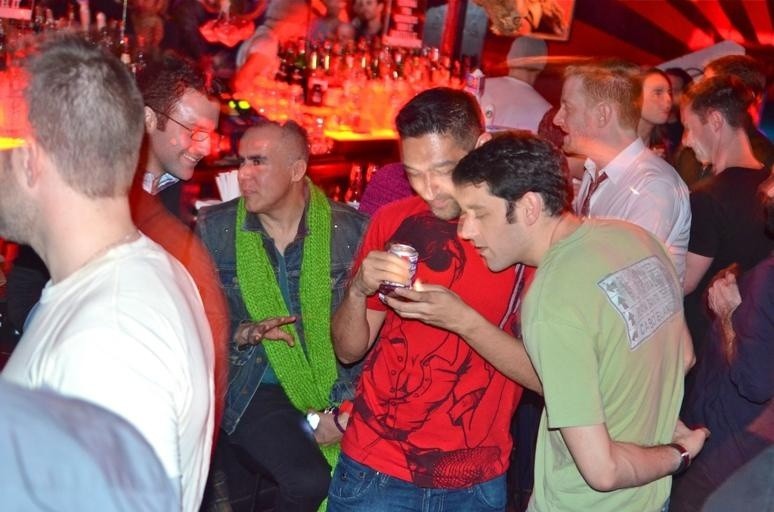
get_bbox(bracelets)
[333,414,346,433]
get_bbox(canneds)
[378,244,420,305]
[302,407,335,435]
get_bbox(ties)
[583,174,608,215]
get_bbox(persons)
[552,57,692,290]
[328,87,545,509]
[637,53,772,512]
[506,108,588,506]
[357,162,416,215]
[2,29,373,509]
[451,131,710,511]
[115,0,397,104]
[479,34,553,132]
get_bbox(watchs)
[666,443,689,475]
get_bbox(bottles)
[13,5,148,79]
[234,34,468,143]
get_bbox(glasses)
[146,104,210,143]
